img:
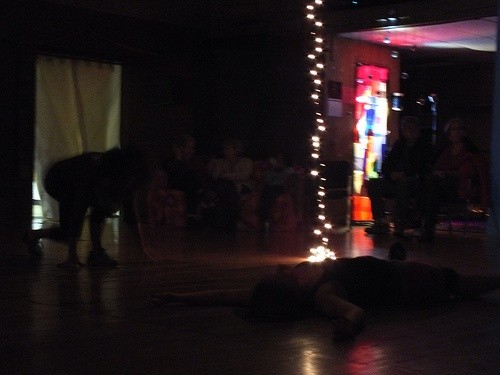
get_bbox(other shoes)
[364,222,390,234]
[87,248,121,268]
[21,229,44,257]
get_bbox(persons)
[46,145,162,269]
[365,116,433,237]
[427,120,479,229]
[147,255,500,339]
